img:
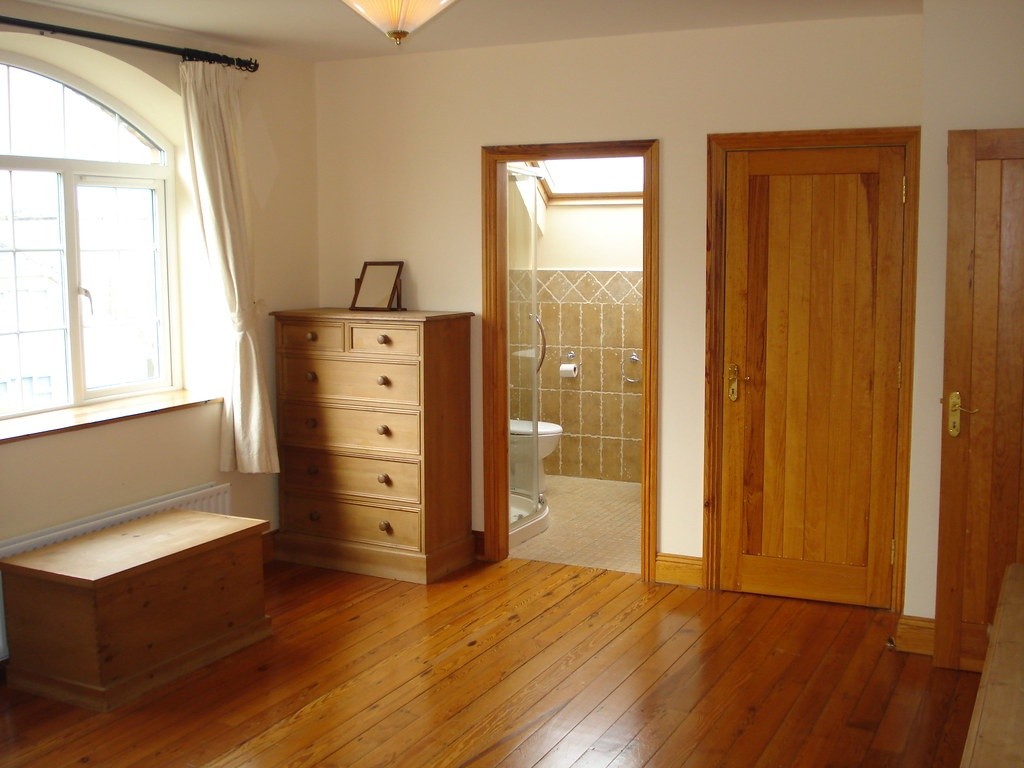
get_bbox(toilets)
[509,418,563,497]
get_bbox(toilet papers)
[559,363,578,378]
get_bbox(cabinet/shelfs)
[268,306,476,585]
[0,510,276,712]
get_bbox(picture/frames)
[349,261,408,311]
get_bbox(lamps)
[342,0,456,45]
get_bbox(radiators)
[1,482,233,661]
